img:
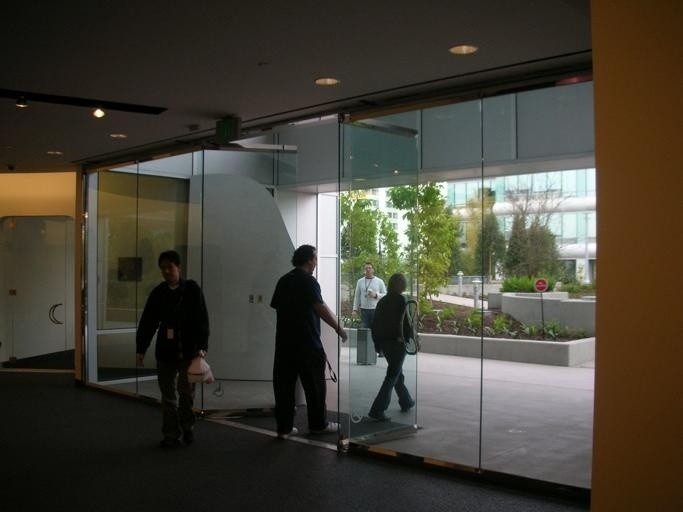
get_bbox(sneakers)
[400,399,417,413]
[182,431,197,445]
[277,426,299,441]
[368,413,392,422]
[159,427,182,448]
[309,421,342,434]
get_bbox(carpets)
[225,397,412,445]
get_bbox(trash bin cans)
[357,327,377,366]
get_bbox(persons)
[367,272,415,424]
[134,251,212,445]
[350,262,388,356]
[270,245,347,435]
